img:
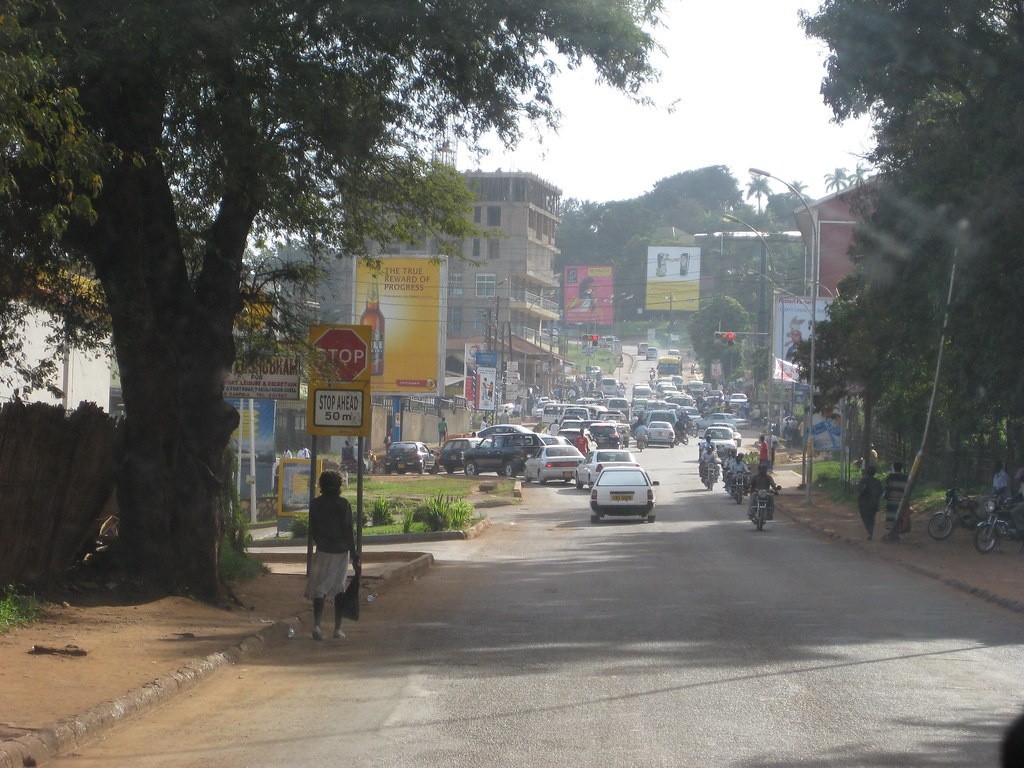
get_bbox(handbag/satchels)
[898,504,911,532]
[860,478,867,497]
[339,571,360,621]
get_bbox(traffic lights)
[580,333,599,342]
[714,331,735,340]
[592,341,598,346]
[727,339,735,345]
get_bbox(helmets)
[706,434,711,441]
[759,464,768,473]
[728,448,743,460]
[706,445,714,453]
[1014,468,1024,482]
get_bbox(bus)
[655,355,683,379]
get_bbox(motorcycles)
[342,450,385,476]
[742,483,782,531]
[973,494,1024,555]
[724,465,752,505]
[700,458,721,491]
[927,475,997,541]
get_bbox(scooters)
[649,371,655,380]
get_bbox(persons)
[650,367,656,378]
[283,446,292,459]
[576,429,589,457]
[744,464,779,524]
[759,435,768,463]
[499,409,509,424]
[514,403,522,417]
[482,377,493,401]
[698,434,749,496]
[550,419,560,436]
[341,440,356,463]
[527,381,594,404]
[696,388,751,418]
[675,410,692,435]
[438,417,448,446]
[309,470,359,638]
[991,461,1009,495]
[1014,467,1024,498]
[297,444,312,459]
[856,463,882,541]
[884,462,909,541]
[786,329,806,361]
[756,413,782,448]
[631,410,648,446]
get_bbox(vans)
[599,336,614,348]
[637,342,650,356]
[646,347,658,360]
[668,349,679,355]
[438,437,497,474]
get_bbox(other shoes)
[312,625,322,641]
[333,630,346,639]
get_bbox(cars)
[385,441,439,475]
[589,466,660,524]
[711,422,741,447]
[531,375,748,450]
[575,449,639,490]
[698,427,738,462]
[523,445,586,485]
[469,424,534,440]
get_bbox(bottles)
[367,594,374,602]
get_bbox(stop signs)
[311,328,368,384]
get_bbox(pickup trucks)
[460,433,574,478]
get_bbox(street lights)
[562,288,635,384]
[522,288,556,422]
[720,213,775,464]
[748,167,819,504]
